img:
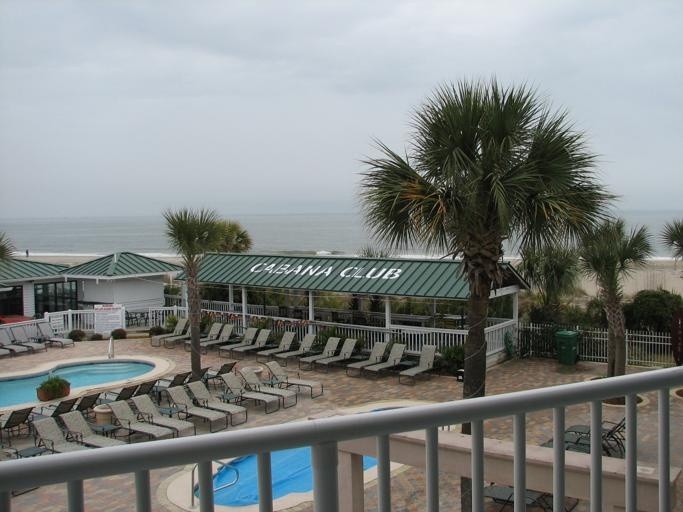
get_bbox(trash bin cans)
[457,369,463,382]
[554,330,578,365]
[93,404,112,425]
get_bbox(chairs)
[0,322,75,359]
[125,305,170,327]
[482,416,626,512]
[0,360,324,497]
[150,318,437,388]
